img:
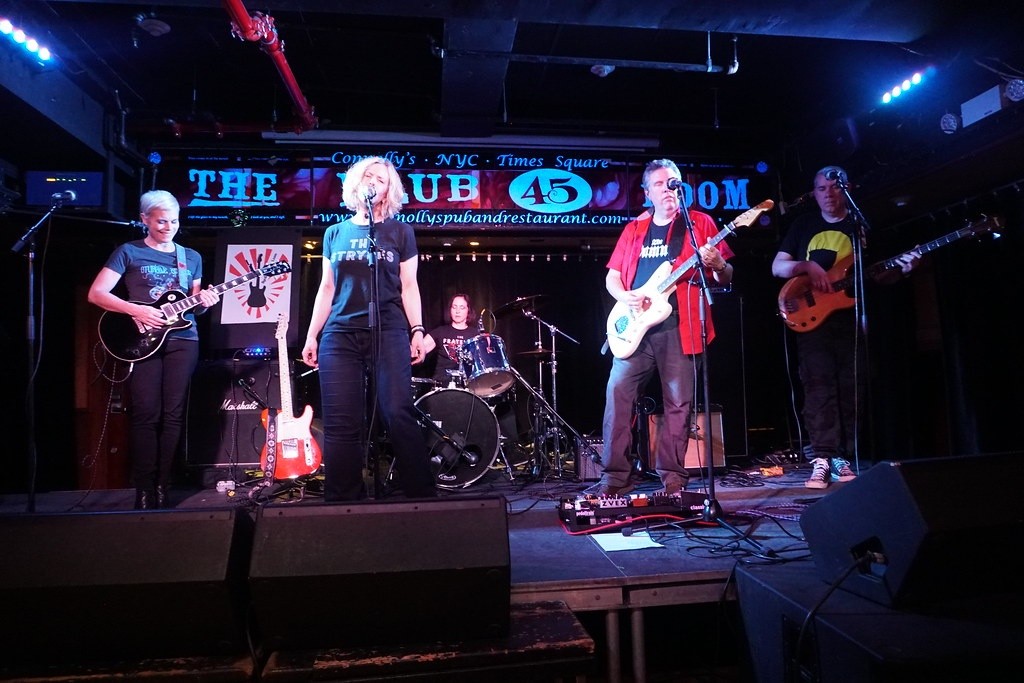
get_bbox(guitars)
[606,198,774,359]
[98,260,295,363]
[259,313,322,480]
[779,213,1012,333]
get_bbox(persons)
[771,166,923,489]
[422,292,482,382]
[87,190,221,512]
[302,157,440,504]
[593,156,736,500]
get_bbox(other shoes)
[134,480,173,511]
[589,483,634,497]
[667,490,681,506]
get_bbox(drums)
[414,389,500,488]
[455,331,515,398]
[410,375,443,404]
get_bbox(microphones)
[467,452,480,464]
[667,177,682,190]
[477,313,485,333]
[590,453,602,463]
[365,187,376,199]
[52,190,76,202]
[689,428,700,433]
[238,376,255,384]
[825,169,843,180]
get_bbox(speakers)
[181,357,298,471]
[637,293,748,458]
[245,492,511,651]
[799,451,1024,614]
[637,403,726,476]
[0,505,257,675]
[575,435,607,481]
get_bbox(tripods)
[516,309,580,488]
[621,185,775,557]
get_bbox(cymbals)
[502,294,545,307]
[516,347,563,360]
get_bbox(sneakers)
[805,458,830,489]
[828,457,857,483]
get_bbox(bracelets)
[411,325,426,335]
[717,262,727,274]
